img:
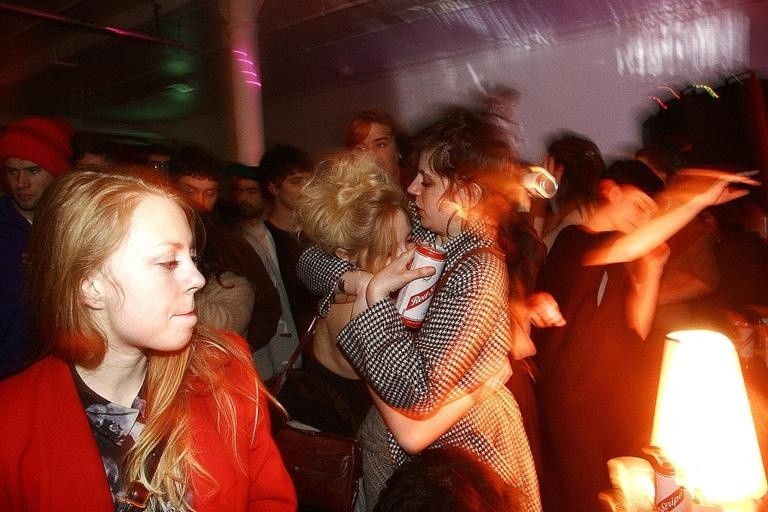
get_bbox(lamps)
[651,329,768,512]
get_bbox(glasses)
[150,160,171,170]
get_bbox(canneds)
[655,469,687,512]
[394,242,446,331]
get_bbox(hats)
[608,158,667,207]
[0,115,75,176]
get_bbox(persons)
[1,66,768,511]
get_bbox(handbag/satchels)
[277,419,360,510]
